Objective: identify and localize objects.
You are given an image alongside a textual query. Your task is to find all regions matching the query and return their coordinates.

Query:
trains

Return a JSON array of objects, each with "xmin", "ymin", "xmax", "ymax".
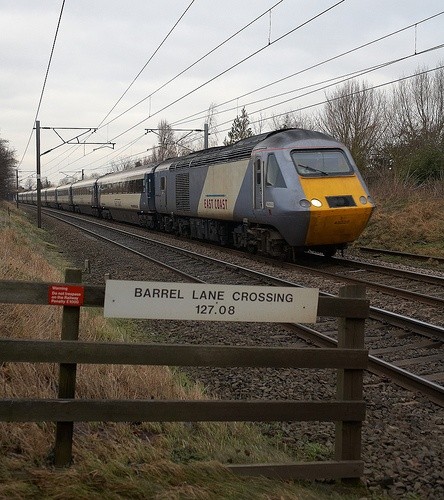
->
[{"xmin": 14, "ymin": 125, "xmax": 374, "ymax": 261}]
